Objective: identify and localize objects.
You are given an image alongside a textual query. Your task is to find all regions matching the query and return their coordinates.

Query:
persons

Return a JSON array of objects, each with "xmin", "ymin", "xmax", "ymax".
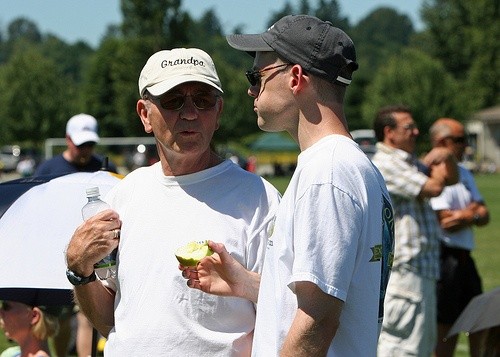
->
[
  {"xmin": 68, "ymin": 288, "xmax": 97, "ymax": 357},
  {"xmin": 66, "ymin": 47, "xmax": 283, "ymax": 357},
  {"xmin": 371, "ymin": 107, "xmax": 459, "ymax": 357},
  {"xmin": 178, "ymin": 14, "xmax": 394, "ymax": 357},
  {"xmin": 34, "ymin": 113, "xmax": 115, "ymax": 175},
  {"xmin": 0, "ymin": 288, "xmax": 62, "ymax": 357},
  {"xmin": 427, "ymin": 117, "xmax": 489, "ymax": 357}
]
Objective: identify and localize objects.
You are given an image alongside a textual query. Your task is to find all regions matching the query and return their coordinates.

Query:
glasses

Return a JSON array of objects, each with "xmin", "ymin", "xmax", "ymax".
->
[
  {"xmin": 244, "ymin": 63, "xmax": 308, "ymax": 86},
  {"xmin": 143, "ymin": 92, "xmax": 220, "ymax": 111}
]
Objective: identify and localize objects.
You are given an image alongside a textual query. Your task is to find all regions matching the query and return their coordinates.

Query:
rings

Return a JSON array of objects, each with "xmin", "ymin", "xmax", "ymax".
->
[{"xmin": 113, "ymin": 229, "xmax": 120, "ymax": 239}]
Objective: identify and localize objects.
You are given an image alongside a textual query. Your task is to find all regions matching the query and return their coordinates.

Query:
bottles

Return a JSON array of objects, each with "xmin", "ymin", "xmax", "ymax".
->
[{"xmin": 81, "ymin": 187, "xmax": 116, "ymax": 280}]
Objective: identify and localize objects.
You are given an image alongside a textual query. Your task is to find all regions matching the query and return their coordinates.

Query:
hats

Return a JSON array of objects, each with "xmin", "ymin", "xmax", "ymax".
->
[
  {"xmin": 226, "ymin": 14, "xmax": 359, "ymax": 86},
  {"xmin": 65, "ymin": 113, "xmax": 100, "ymax": 147},
  {"xmin": 138, "ymin": 47, "xmax": 224, "ymax": 99}
]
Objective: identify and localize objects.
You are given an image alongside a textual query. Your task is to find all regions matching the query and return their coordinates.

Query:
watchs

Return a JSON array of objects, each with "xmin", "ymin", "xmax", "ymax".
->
[
  {"xmin": 473, "ymin": 211, "xmax": 480, "ymax": 225},
  {"xmin": 65, "ymin": 267, "xmax": 96, "ymax": 286}
]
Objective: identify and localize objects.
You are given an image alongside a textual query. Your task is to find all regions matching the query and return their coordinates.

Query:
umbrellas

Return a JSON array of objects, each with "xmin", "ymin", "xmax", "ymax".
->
[
  {"xmin": 0, "ymin": 169, "xmax": 125, "ymax": 288},
  {"xmin": 443, "ymin": 287, "xmax": 500, "ymax": 342}
]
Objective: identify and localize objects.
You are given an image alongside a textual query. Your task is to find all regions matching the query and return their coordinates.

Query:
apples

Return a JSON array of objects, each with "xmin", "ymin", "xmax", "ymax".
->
[{"xmin": 174, "ymin": 242, "xmax": 214, "ymax": 267}]
